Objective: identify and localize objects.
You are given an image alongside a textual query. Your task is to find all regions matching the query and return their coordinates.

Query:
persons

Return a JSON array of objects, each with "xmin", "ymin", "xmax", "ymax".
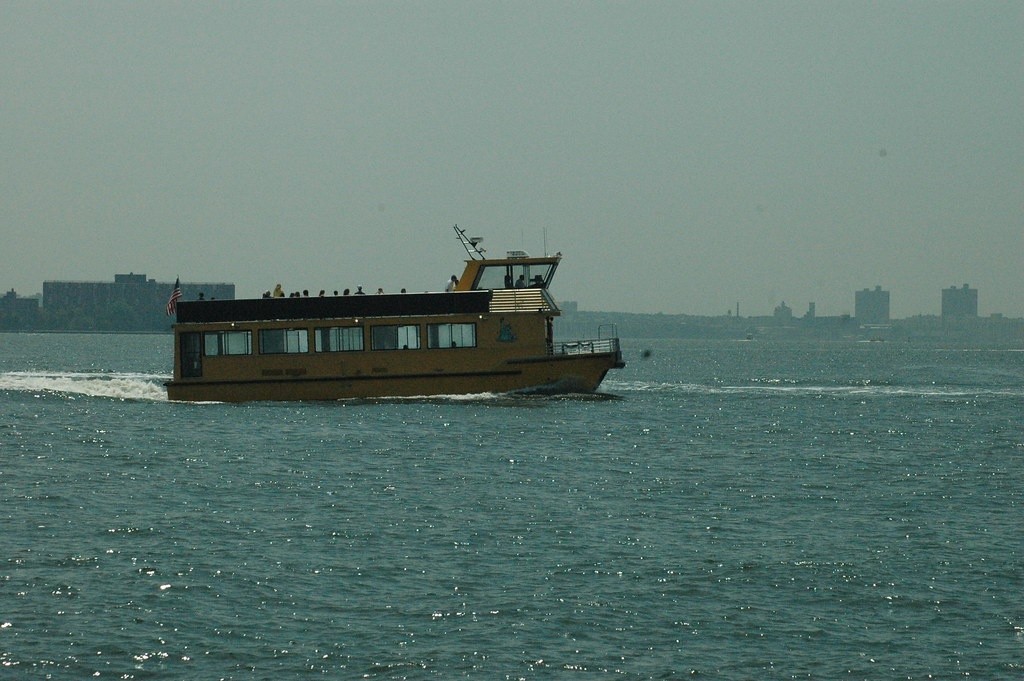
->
[
  {"xmin": 401, "ymin": 288, "xmax": 406, "ymax": 293},
  {"xmin": 515, "ymin": 275, "xmax": 525, "ymax": 288},
  {"xmin": 262, "ymin": 284, "xmax": 285, "ymax": 298},
  {"xmin": 529, "ymin": 275, "xmax": 544, "ymax": 288},
  {"xmin": 303, "ymin": 290, "xmax": 309, "ymax": 297},
  {"xmin": 334, "ymin": 290, "xmax": 338, "ymax": 296},
  {"xmin": 319, "ymin": 290, "xmax": 325, "ymax": 296},
  {"xmin": 289, "ymin": 291, "xmax": 300, "ymax": 298},
  {"xmin": 344, "ymin": 289, "xmax": 350, "ymax": 295},
  {"xmin": 354, "ymin": 286, "xmax": 365, "ymax": 295},
  {"xmin": 375, "ymin": 288, "xmax": 384, "ymax": 294},
  {"xmin": 445, "ymin": 275, "xmax": 459, "ymax": 292},
  {"xmin": 199, "ymin": 292, "xmax": 205, "ymax": 300},
  {"xmin": 505, "ymin": 275, "xmax": 513, "ymax": 288},
  {"xmin": 211, "ymin": 297, "xmax": 215, "ymax": 300},
  {"xmin": 451, "ymin": 341, "xmax": 457, "ymax": 347},
  {"xmin": 403, "ymin": 345, "xmax": 407, "ymax": 349}
]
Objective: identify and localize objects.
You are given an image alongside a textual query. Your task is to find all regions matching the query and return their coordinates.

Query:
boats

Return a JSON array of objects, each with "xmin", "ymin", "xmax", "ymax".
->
[{"xmin": 163, "ymin": 223, "xmax": 625, "ymax": 401}]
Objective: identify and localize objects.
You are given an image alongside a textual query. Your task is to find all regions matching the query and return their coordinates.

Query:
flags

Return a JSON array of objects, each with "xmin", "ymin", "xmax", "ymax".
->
[{"xmin": 166, "ymin": 276, "xmax": 182, "ymax": 316}]
